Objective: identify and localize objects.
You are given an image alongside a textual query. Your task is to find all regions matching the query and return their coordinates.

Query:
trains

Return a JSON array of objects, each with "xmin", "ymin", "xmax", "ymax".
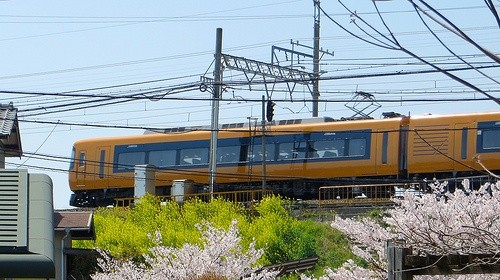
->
[{"xmin": 68, "ymin": 113, "xmax": 500, "ymax": 210}]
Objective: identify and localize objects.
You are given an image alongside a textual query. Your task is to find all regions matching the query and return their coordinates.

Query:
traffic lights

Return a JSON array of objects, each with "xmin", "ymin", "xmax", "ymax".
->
[{"xmin": 267, "ymin": 99, "xmax": 274, "ymax": 122}]
